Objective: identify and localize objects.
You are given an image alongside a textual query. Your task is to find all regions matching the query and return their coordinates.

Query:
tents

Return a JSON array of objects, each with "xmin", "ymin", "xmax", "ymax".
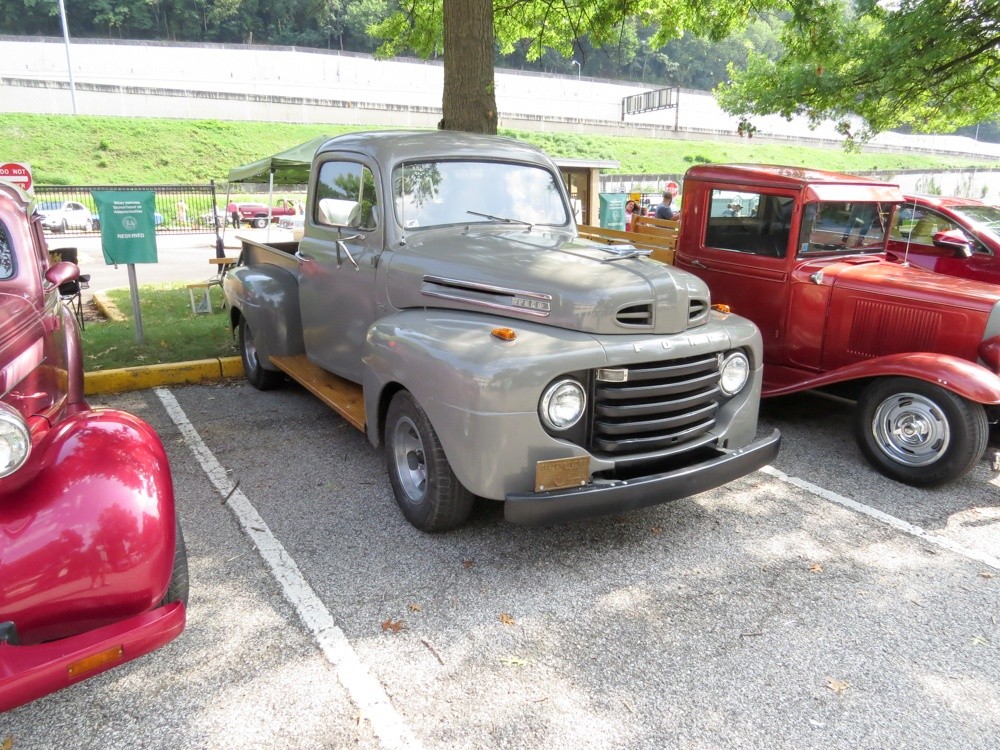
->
[{"xmin": 223, "ymin": 134, "xmax": 332, "ymax": 243}]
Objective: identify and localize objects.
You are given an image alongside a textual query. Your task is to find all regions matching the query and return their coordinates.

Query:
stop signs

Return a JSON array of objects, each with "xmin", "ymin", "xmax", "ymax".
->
[
  {"xmin": 0, "ymin": 161, "xmax": 35, "ymax": 196},
  {"xmin": 665, "ymin": 182, "xmax": 678, "ymax": 197}
]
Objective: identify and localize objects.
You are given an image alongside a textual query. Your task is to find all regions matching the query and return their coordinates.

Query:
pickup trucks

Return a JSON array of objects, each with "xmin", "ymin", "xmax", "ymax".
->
[
  {"xmin": 239, "ymin": 199, "xmax": 305, "ymax": 228},
  {"xmin": 223, "ymin": 129, "xmax": 782, "ymax": 537},
  {"xmin": 575, "ymin": 165, "xmax": 1000, "ymax": 491}
]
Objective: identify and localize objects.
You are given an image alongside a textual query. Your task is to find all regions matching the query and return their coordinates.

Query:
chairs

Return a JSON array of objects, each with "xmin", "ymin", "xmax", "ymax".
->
[
  {"xmin": 186, "ymin": 255, "xmax": 242, "ymax": 315},
  {"xmin": 48, "ymin": 248, "xmax": 90, "ymax": 331}
]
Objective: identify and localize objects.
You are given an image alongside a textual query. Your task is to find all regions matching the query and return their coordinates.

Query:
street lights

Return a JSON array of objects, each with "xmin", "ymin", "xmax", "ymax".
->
[{"xmin": 571, "ymin": 59, "xmax": 581, "ymax": 81}]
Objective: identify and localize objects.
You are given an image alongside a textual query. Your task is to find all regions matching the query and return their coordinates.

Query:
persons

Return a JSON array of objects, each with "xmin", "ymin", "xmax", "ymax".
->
[
  {"xmin": 625, "ymin": 191, "xmax": 681, "ymax": 232},
  {"xmin": 213, "ymin": 199, "xmax": 220, "ymax": 222},
  {"xmin": 228, "ymin": 200, "xmax": 240, "ymax": 229},
  {"xmin": 721, "ymin": 196, "xmax": 758, "ymax": 217},
  {"xmin": 175, "ymin": 198, "xmax": 189, "ymax": 223},
  {"xmin": 831, "ymin": 201, "xmax": 876, "ymax": 248}
]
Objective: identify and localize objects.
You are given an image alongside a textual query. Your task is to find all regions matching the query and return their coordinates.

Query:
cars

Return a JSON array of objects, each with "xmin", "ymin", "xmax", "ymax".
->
[
  {"xmin": 197, "ymin": 202, "xmax": 265, "ymax": 228},
  {"xmin": 886, "ymin": 195, "xmax": 1000, "ymax": 286},
  {"xmin": 0, "ymin": 184, "xmax": 193, "ymax": 717},
  {"xmin": 34, "ymin": 201, "xmax": 93, "ymax": 233}
]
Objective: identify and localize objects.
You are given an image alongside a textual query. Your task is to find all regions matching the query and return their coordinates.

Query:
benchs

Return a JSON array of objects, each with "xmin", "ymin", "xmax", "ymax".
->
[{"xmin": 709, "ymin": 227, "xmax": 801, "ymax": 257}]
